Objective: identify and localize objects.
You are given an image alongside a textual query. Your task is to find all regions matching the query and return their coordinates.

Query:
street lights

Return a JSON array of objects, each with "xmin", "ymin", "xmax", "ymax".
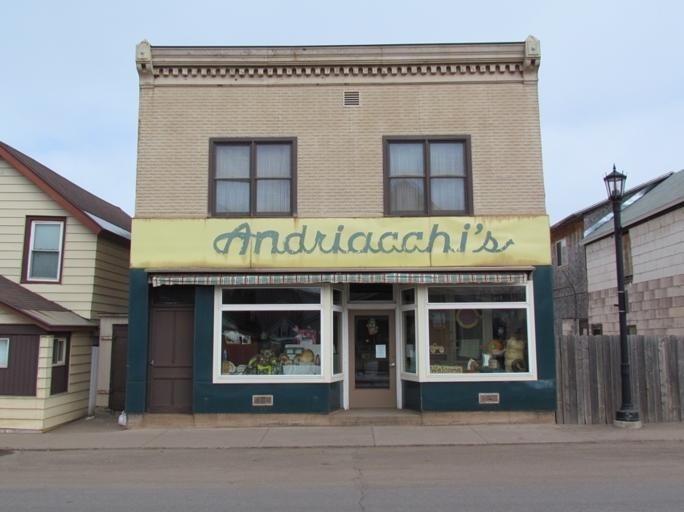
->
[{"xmin": 603, "ymin": 164, "xmax": 641, "ymax": 430}]
